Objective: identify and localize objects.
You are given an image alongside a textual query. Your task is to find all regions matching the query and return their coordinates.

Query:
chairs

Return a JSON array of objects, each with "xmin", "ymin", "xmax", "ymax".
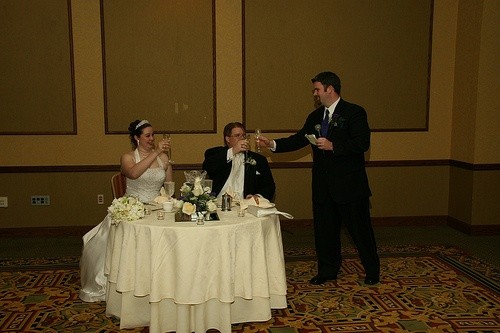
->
[{"xmin": 111, "ymin": 172, "xmax": 125, "ymax": 199}]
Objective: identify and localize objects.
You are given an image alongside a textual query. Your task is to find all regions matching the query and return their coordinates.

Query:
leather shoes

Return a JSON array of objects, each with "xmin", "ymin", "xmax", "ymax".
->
[
  {"xmin": 309, "ymin": 274, "xmax": 338, "ymax": 285},
  {"xmin": 364, "ymin": 272, "xmax": 379, "ymax": 285}
]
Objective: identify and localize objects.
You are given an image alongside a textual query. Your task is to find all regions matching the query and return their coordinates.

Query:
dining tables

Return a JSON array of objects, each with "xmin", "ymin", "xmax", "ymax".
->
[{"xmin": 102, "ymin": 194, "xmax": 288, "ymax": 333}]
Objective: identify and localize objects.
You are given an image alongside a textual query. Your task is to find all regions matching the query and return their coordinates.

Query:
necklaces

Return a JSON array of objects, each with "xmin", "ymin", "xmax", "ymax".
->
[{"xmin": 137, "ymin": 149, "xmax": 148, "ymax": 158}]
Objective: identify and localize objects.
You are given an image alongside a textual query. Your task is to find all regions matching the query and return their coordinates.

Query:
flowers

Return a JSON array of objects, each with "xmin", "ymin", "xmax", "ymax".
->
[
  {"xmin": 106, "ymin": 194, "xmax": 147, "ymax": 226},
  {"xmin": 175, "ymin": 178, "xmax": 217, "ymax": 216}
]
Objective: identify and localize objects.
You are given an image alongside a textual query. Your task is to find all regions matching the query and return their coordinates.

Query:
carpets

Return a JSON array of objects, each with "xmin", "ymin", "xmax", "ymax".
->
[{"xmin": 0, "ymin": 244, "xmax": 500, "ymax": 333}]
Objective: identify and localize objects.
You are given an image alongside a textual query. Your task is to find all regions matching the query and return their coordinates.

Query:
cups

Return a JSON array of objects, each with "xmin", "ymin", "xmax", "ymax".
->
[
  {"xmin": 222, "ymin": 193, "xmax": 232, "ymax": 212},
  {"xmin": 144, "ymin": 206, "xmax": 152, "ymax": 215},
  {"xmin": 238, "ymin": 209, "xmax": 245, "ymax": 217},
  {"xmin": 254, "ymin": 128, "xmax": 263, "ymax": 154},
  {"xmin": 163, "ymin": 202, "xmax": 173, "ymax": 211},
  {"xmin": 156, "ymin": 211, "xmax": 165, "ymax": 220},
  {"xmin": 202, "ymin": 180, "xmax": 213, "ymax": 192},
  {"xmin": 196, "ymin": 218, "xmax": 203, "ymax": 225}
]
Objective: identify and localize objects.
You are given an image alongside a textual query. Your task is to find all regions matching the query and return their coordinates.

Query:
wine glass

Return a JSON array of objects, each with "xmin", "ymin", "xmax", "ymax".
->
[
  {"xmin": 164, "ymin": 182, "xmax": 175, "ymax": 201},
  {"xmin": 239, "ymin": 134, "xmax": 253, "ymax": 164},
  {"xmin": 163, "ymin": 133, "xmax": 175, "ymax": 164}
]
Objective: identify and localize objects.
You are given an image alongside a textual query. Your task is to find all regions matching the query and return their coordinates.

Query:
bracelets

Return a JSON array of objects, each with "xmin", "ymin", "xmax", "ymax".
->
[{"xmin": 148, "ymin": 148, "xmax": 158, "ymax": 161}]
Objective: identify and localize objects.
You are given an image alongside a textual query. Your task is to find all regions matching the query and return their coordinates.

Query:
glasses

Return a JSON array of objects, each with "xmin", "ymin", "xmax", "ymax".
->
[{"xmin": 230, "ymin": 135, "xmax": 247, "ymax": 140}]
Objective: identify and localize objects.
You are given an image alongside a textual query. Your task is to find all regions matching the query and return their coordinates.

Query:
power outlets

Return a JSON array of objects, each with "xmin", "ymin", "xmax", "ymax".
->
[
  {"xmin": 97, "ymin": 194, "xmax": 104, "ymax": 205},
  {"xmin": 30, "ymin": 195, "xmax": 50, "ymax": 205},
  {"xmin": 0, "ymin": 197, "xmax": 9, "ymax": 208}
]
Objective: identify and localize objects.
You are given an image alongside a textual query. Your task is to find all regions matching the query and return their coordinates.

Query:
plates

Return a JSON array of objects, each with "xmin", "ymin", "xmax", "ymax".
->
[{"xmin": 158, "ymin": 210, "xmax": 177, "ymax": 213}]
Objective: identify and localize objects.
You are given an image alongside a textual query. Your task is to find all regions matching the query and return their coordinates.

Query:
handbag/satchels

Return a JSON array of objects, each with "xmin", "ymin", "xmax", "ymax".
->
[{"xmin": 248, "ymin": 204, "xmax": 296, "ymax": 220}]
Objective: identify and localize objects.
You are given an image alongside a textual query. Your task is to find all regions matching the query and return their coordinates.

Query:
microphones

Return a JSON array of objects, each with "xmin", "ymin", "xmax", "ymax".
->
[{"xmin": 314, "ymin": 124, "xmax": 326, "ymax": 154}]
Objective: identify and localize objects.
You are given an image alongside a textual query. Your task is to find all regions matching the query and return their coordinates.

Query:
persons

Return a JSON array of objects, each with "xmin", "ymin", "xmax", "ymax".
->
[
  {"xmin": 120, "ymin": 119, "xmax": 172, "ymax": 202},
  {"xmin": 202, "ymin": 122, "xmax": 276, "ymax": 202},
  {"xmin": 256, "ymin": 72, "xmax": 378, "ymax": 285}
]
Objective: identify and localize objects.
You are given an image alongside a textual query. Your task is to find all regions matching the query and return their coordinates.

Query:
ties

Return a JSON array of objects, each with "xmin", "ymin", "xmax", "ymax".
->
[{"xmin": 322, "ymin": 109, "xmax": 329, "ymax": 136}]
